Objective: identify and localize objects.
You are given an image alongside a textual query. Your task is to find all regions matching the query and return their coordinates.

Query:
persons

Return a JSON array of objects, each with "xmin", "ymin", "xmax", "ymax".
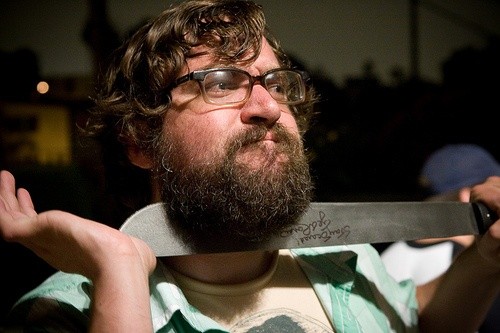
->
[
  {"xmin": 380, "ymin": 144, "xmax": 500, "ymax": 333},
  {"xmin": 0, "ymin": 0, "xmax": 500, "ymax": 333}
]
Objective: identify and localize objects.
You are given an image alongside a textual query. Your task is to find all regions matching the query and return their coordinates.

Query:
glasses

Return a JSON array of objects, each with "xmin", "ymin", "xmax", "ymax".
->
[{"xmin": 158, "ymin": 67, "xmax": 307, "ymax": 104}]
